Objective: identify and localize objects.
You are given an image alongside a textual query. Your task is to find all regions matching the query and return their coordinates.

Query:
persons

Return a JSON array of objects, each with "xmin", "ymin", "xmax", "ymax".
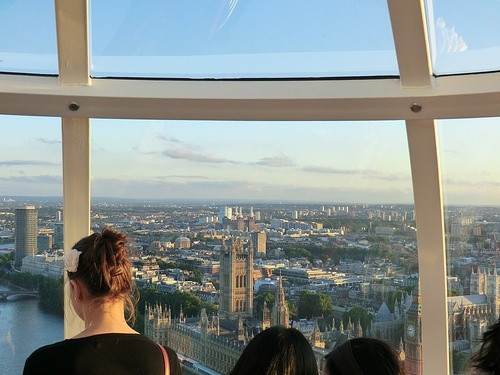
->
[
  {"xmin": 323, "ymin": 336, "xmax": 405, "ymax": 374},
  {"xmin": 21, "ymin": 227, "xmax": 183, "ymax": 375},
  {"xmin": 229, "ymin": 325, "xmax": 320, "ymax": 374}
]
